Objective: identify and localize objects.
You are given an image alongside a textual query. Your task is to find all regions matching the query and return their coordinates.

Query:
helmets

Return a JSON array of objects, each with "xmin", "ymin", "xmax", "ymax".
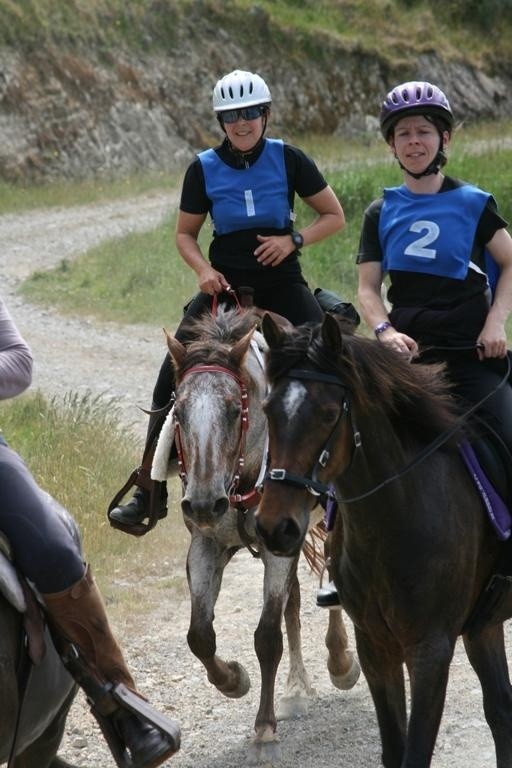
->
[
  {"xmin": 380, "ymin": 80, "xmax": 454, "ymax": 144},
  {"xmin": 213, "ymin": 70, "xmax": 272, "ymax": 112}
]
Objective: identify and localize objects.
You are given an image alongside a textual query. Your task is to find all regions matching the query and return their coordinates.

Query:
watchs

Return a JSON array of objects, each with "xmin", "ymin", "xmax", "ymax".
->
[
  {"xmin": 374, "ymin": 322, "xmax": 393, "ymax": 336},
  {"xmin": 289, "ymin": 231, "xmax": 304, "ymax": 251}
]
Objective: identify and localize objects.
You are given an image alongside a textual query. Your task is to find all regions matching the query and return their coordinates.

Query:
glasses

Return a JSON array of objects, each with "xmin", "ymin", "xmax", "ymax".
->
[{"xmin": 222, "ymin": 108, "xmax": 266, "ymax": 123}]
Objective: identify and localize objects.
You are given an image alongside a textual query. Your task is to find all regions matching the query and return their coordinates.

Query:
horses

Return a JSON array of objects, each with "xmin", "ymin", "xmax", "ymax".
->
[
  {"xmin": 253, "ymin": 310, "xmax": 511, "ymax": 768},
  {"xmin": 0, "ymin": 515, "xmax": 83, "ymax": 767},
  {"xmin": 153, "ymin": 301, "xmax": 366, "ymax": 768}
]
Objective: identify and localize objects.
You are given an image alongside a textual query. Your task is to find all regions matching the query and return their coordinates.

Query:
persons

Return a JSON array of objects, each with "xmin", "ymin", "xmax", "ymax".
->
[
  {"xmin": 0, "ymin": 296, "xmax": 182, "ymax": 768},
  {"xmin": 315, "ymin": 82, "xmax": 512, "ymax": 612},
  {"xmin": 107, "ymin": 70, "xmax": 344, "ymax": 536}
]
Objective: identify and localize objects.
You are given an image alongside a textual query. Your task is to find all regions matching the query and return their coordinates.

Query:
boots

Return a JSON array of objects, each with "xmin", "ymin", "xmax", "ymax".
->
[
  {"xmin": 43, "ymin": 560, "xmax": 173, "ymax": 767},
  {"xmin": 315, "ymin": 580, "xmax": 341, "ymax": 607},
  {"xmin": 109, "ymin": 401, "xmax": 175, "ymax": 525}
]
